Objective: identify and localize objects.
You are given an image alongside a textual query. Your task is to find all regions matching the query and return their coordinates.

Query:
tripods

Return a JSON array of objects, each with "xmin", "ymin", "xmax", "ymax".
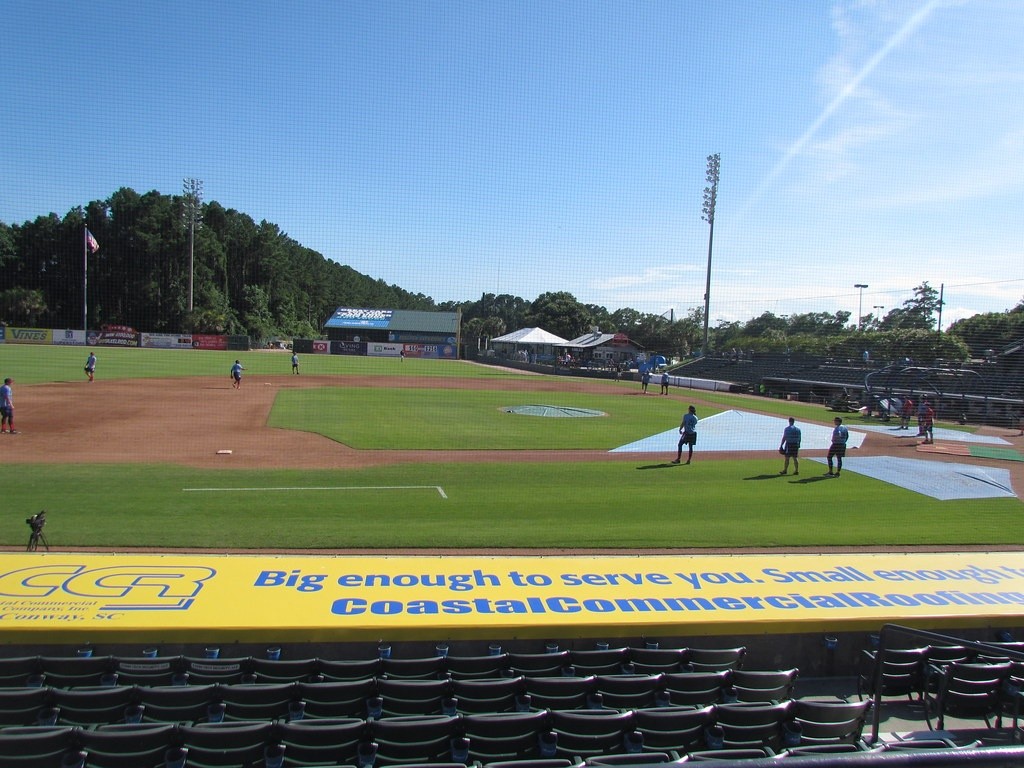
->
[{"xmin": 26, "ymin": 532, "xmax": 49, "ymax": 552}]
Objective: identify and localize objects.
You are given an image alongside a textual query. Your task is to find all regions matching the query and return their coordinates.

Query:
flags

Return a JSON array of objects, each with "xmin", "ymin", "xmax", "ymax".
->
[{"xmin": 87, "ymin": 230, "xmax": 99, "ymax": 253}]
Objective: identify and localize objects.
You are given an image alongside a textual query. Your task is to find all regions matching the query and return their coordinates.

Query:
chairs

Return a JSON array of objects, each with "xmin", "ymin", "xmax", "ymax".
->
[{"xmin": 0, "ymin": 642, "xmax": 1024, "ymax": 768}]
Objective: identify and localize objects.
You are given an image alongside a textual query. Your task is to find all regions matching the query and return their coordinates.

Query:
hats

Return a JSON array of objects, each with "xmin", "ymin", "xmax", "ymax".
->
[{"xmin": 832, "ymin": 416, "xmax": 843, "ymax": 423}]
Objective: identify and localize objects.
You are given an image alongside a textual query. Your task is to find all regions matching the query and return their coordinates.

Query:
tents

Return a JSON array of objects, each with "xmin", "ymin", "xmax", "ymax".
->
[{"xmin": 490, "ymin": 327, "xmax": 570, "ymax": 359}]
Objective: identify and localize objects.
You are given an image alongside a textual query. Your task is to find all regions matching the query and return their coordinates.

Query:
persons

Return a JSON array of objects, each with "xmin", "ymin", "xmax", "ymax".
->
[
  {"xmin": 615, "ymin": 365, "xmax": 623, "ymax": 382},
  {"xmin": 918, "ymin": 393, "xmax": 934, "ymax": 444},
  {"xmin": 898, "ymin": 395, "xmax": 913, "ymax": 430},
  {"xmin": 497, "ymin": 350, "xmax": 629, "ymax": 371},
  {"xmin": 725, "ymin": 347, "xmax": 744, "ymax": 364},
  {"xmin": 84, "ymin": 352, "xmax": 96, "ymax": 383},
  {"xmin": 863, "ymin": 347, "xmax": 869, "ymax": 369},
  {"xmin": 231, "ymin": 360, "xmax": 248, "ymax": 389},
  {"xmin": 642, "ymin": 370, "xmax": 652, "ymax": 394},
  {"xmin": 754, "ymin": 384, "xmax": 759, "ymax": 395},
  {"xmin": 292, "ymin": 351, "xmax": 299, "ymax": 374},
  {"xmin": 824, "ymin": 417, "xmax": 848, "ymax": 477},
  {"xmin": 779, "ymin": 417, "xmax": 801, "ymax": 475},
  {"xmin": 660, "ymin": 370, "xmax": 669, "ymax": 395},
  {"xmin": 671, "ymin": 406, "xmax": 698, "ymax": 464},
  {"xmin": 400, "ymin": 349, "xmax": 405, "ymax": 361},
  {"xmin": 759, "ymin": 383, "xmax": 765, "ymax": 397},
  {"xmin": 0, "ymin": 377, "xmax": 21, "ymax": 434}
]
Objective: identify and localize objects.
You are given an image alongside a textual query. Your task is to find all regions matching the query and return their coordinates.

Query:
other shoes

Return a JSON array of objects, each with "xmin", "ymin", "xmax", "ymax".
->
[
  {"xmin": 685, "ymin": 460, "xmax": 690, "ymax": 464},
  {"xmin": 236, "ymin": 386, "xmax": 241, "ymax": 390},
  {"xmin": 671, "ymin": 459, "xmax": 681, "ymax": 464},
  {"xmin": 780, "ymin": 471, "xmax": 787, "ymax": 474},
  {"xmin": 10, "ymin": 430, "xmax": 21, "ymax": 434},
  {"xmin": 905, "ymin": 427, "xmax": 908, "ymax": 430},
  {"xmin": 644, "ymin": 391, "xmax": 648, "ymax": 394},
  {"xmin": 922, "ymin": 439, "xmax": 928, "ymax": 443},
  {"xmin": 927, "ymin": 441, "xmax": 933, "ymax": 445},
  {"xmin": 823, "ymin": 472, "xmax": 832, "ymax": 476},
  {"xmin": 232, "ymin": 383, "xmax": 236, "ymax": 387},
  {"xmin": 88, "ymin": 379, "xmax": 94, "ymax": 381},
  {"xmin": 2, "ymin": 429, "xmax": 9, "ymax": 432},
  {"xmin": 898, "ymin": 427, "xmax": 903, "ymax": 429},
  {"xmin": 660, "ymin": 393, "xmax": 663, "ymax": 394},
  {"xmin": 792, "ymin": 472, "xmax": 799, "ymax": 475},
  {"xmin": 665, "ymin": 393, "xmax": 668, "ymax": 396},
  {"xmin": 834, "ymin": 472, "xmax": 840, "ymax": 477},
  {"xmin": 297, "ymin": 372, "xmax": 299, "ymax": 375},
  {"xmin": 918, "ymin": 433, "xmax": 925, "ymax": 436}
]
